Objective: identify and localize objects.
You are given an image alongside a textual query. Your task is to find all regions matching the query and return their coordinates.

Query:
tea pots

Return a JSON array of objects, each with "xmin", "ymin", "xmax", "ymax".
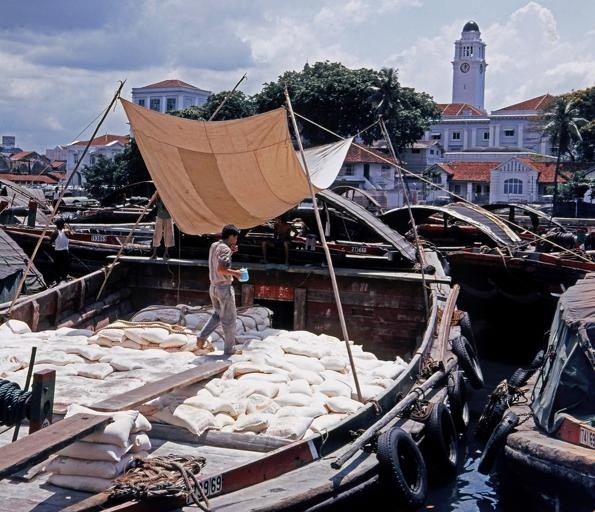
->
[{"xmin": 237, "ymin": 268, "xmax": 251, "ymax": 283}]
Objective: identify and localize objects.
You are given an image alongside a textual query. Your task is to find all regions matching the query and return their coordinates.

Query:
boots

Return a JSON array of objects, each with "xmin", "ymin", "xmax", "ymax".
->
[
  {"xmin": 163, "ymin": 247, "xmax": 169, "ymax": 260},
  {"xmin": 150, "ymin": 247, "xmax": 158, "ymax": 260}
]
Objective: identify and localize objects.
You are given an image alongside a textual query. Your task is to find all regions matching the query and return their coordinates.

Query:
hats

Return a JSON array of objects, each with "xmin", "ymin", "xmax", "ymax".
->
[{"xmin": 54, "ymin": 218, "xmax": 65, "ymax": 224}]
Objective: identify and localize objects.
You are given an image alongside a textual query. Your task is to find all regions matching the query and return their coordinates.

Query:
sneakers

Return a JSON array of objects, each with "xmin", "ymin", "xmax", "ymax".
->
[{"xmin": 224, "ymin": 351, "xmax": 242, "ymax": 354}]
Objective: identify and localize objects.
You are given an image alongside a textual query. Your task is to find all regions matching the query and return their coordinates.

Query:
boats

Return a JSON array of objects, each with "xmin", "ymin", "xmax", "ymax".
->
[
  {"xmin": 0, "ymin": 184, "xmax": 461, "ymax": 512},
  {"xmin": 501, "ymin": 277, "xmax": 595, "ymax": 489}
]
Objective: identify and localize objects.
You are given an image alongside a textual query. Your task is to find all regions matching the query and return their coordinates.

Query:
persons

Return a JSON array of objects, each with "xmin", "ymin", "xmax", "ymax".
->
[
  {"xmin": 148, "ymin": 191, "xmax": 176, "ymax": 262},
  {"xmin": 49, "ymin": 217, "xmax": 72, "ymax": 284},
  {"xmin": 259, "ymin": 214, "xmax": 299, "ymax": 267},
  {"xmin": 26, "ymin": 196, "xmax": 38, "ymax": 228},
  {"xmin": 196, "ymin": 224, "xmax": 244, "ymax": 356}
]
